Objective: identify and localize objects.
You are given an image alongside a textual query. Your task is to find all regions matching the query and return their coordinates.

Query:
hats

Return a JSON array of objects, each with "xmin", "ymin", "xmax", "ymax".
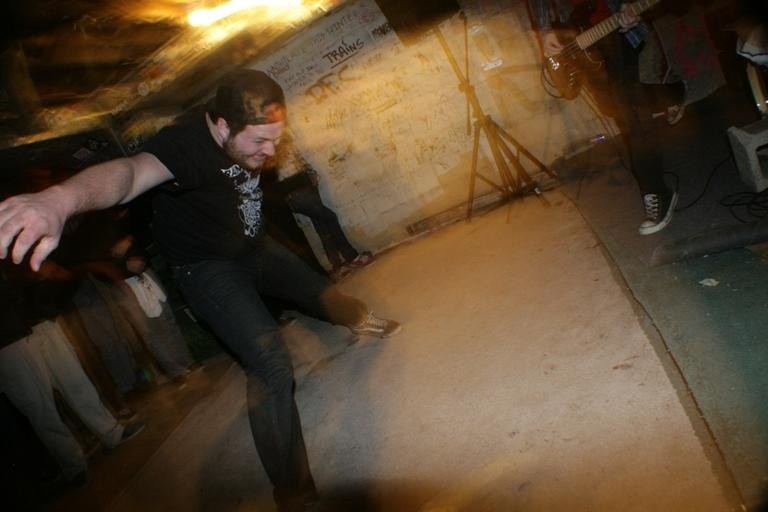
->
[{"xmin": 208, "ymin": 70, "xmax": 286, "ymax": 124}]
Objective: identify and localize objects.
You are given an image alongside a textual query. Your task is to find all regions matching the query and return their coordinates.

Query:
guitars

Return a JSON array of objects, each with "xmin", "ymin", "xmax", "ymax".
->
[{"xmin": 544, "ymin": 0, "xmax": 657, "ymax": 99}]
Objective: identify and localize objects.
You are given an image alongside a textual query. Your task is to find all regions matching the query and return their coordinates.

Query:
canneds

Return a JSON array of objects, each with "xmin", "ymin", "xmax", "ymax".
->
[{"xmin": 590, "ymin": 135, "xmax": 606, "ymax": 142}]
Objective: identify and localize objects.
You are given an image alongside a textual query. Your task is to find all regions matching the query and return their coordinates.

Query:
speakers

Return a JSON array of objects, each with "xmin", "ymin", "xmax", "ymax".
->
[{"xmin": 375, "ymin": 0, "xmax": 461, "ymax": 48}]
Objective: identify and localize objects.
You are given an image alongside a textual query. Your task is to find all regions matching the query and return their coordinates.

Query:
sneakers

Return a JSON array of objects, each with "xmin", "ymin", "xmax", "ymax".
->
[
  {"xmin": 120, "ymin": 425, "xmax": 145, "ymax": 444},
  {"xmin": 118, "ymin": 411, "xmax": 138, "ymax": 423},
  {"xmin": 277, "ymin": 492, "xmax": 328, "ymax": 511},
  {"xmin": 275, "ymin": 311, "xmax": 297, "ymax": 326},
  {"xmin": 335, "ymin": 266, "xmax": 353, "ymax": 280},
  {"xmin": 638, "ymin": 192, "xmax": 679, "ymax": 235},
  {"xmin": 349, "ymin": 254, "xmax": 376, "ymax": 268},
  {"xmin": 351, "ymin": 311, "xmax": 402, "ymax": 338},
  {"xmin": 666, "ymin": 80, "xmax": 689, "ymax": 124}
]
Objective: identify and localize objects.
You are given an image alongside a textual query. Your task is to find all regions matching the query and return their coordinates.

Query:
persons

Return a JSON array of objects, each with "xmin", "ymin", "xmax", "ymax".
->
[
  {"xmin": 526, "ymin": 1, "xmax": 687, "ymax": 237},
  {"xmin": 1, "ymin": 69, "xmax": 404, "ymax": 512}
]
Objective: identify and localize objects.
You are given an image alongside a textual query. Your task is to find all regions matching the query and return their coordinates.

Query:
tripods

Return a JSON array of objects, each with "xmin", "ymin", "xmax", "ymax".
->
[{"xmin": 458, "ymin": 71, "xmax": 564, "ymax": 224}]
